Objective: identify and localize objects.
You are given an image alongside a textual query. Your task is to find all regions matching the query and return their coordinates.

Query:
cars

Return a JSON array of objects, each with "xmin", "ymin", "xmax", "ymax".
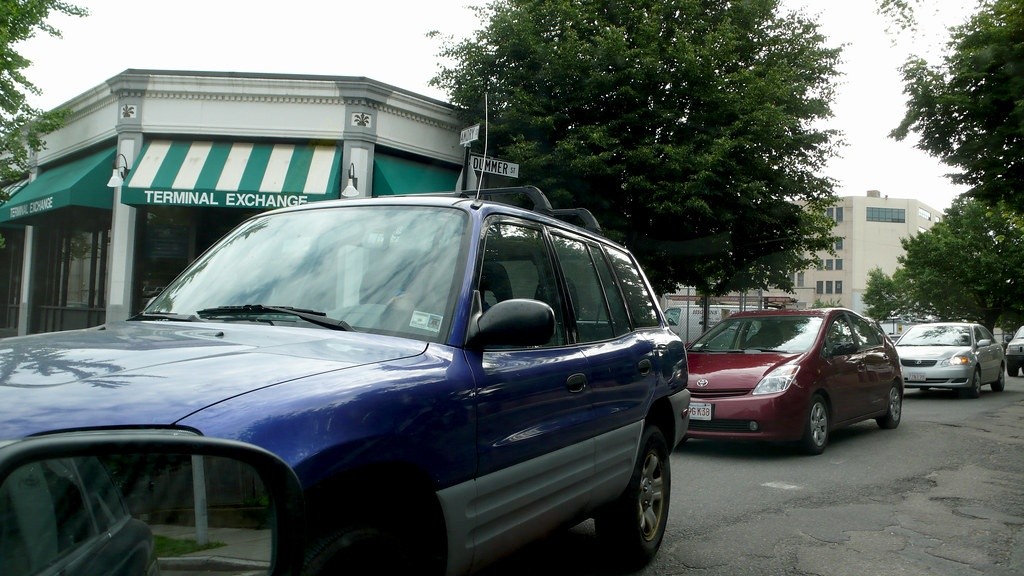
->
[
  {"xmin": 1007, "ymin": 326, "xmax": 1024, "ymax": 377},
  {"xmin": 893, "ymin": 321, "xmax": 1007, "ymax": 398},
  {"xmin": 678, "ymin": 307, "xmax": 905, "ymax": 456}
]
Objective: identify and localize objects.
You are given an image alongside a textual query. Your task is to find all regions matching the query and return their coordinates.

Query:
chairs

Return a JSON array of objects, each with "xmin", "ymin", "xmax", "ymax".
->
[
  {"xmin": 476, "ymin": 261, "xmax": 513, "ymax": 315},
  {"xmin": 534, "ymin": 278, "xmax": 580, "ymax": 350},
  {"xmin": 743, "ymin": 324, "xmax": 783, "ymax": 350}
]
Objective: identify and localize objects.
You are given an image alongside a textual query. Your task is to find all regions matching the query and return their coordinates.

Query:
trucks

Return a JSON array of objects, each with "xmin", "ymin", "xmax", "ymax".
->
[{"xmin": 658, "ymin": 289, "xmax": 800, "ymax": 352}]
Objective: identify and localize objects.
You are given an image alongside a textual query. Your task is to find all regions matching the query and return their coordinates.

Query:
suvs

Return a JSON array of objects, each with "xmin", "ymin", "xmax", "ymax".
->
[{"xmin": 0, "ymin": 183, "xmax": 692, "ymax": 576}]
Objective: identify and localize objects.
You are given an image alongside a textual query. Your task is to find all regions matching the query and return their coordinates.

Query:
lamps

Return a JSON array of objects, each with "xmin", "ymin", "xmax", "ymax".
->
[
  {"xmin": 342, "ymin": 162, "xmax": 359, "ymax": 197},
  {"xmin": 106, "ymin": 153, "xmax": 131, "ymax": 188}
]
